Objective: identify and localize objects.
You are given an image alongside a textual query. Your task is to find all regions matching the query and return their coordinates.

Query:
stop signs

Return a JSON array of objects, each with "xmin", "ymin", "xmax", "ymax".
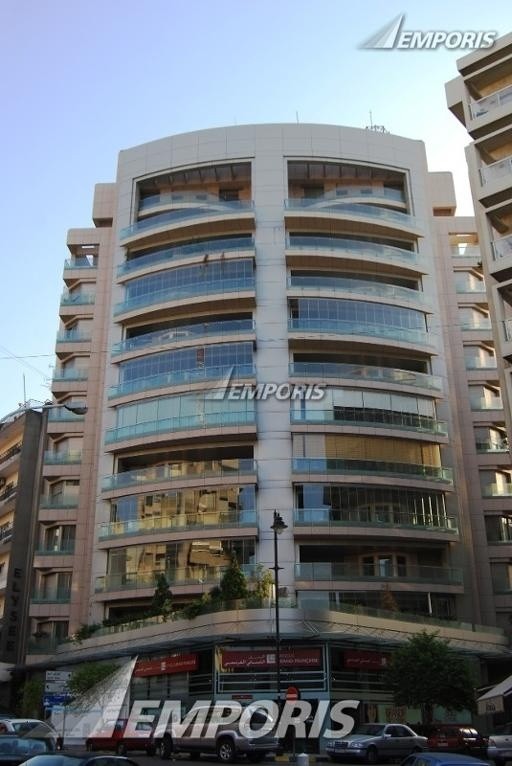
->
[{"xmin": 285, "ymin": 686, "xmax": 299, "ymax": 700}]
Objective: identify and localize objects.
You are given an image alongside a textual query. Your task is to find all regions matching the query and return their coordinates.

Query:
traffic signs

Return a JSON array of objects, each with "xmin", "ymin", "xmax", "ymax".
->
[
  {"xmin": 44, "ymin": 683, "xmax": 71, "ymax": 692},
  {"xmin": 46, "ymin": 671, "xmax": 73, "ymax": 681},
  {"xmin": 43, "ymin": 696, "xmax": 74, "ymax": 705}
]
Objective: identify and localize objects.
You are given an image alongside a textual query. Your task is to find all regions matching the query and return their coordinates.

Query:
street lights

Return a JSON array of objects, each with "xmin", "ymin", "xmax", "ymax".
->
[{"xmin": 269, "ymin": 508, "xmax": 288, "ymax": 699}]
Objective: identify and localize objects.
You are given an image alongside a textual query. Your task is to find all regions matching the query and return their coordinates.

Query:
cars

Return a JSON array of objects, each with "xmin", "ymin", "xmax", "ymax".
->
[{"xmin": 0, "ymin": 717, "xmax": 512, "ymax": 766}]
